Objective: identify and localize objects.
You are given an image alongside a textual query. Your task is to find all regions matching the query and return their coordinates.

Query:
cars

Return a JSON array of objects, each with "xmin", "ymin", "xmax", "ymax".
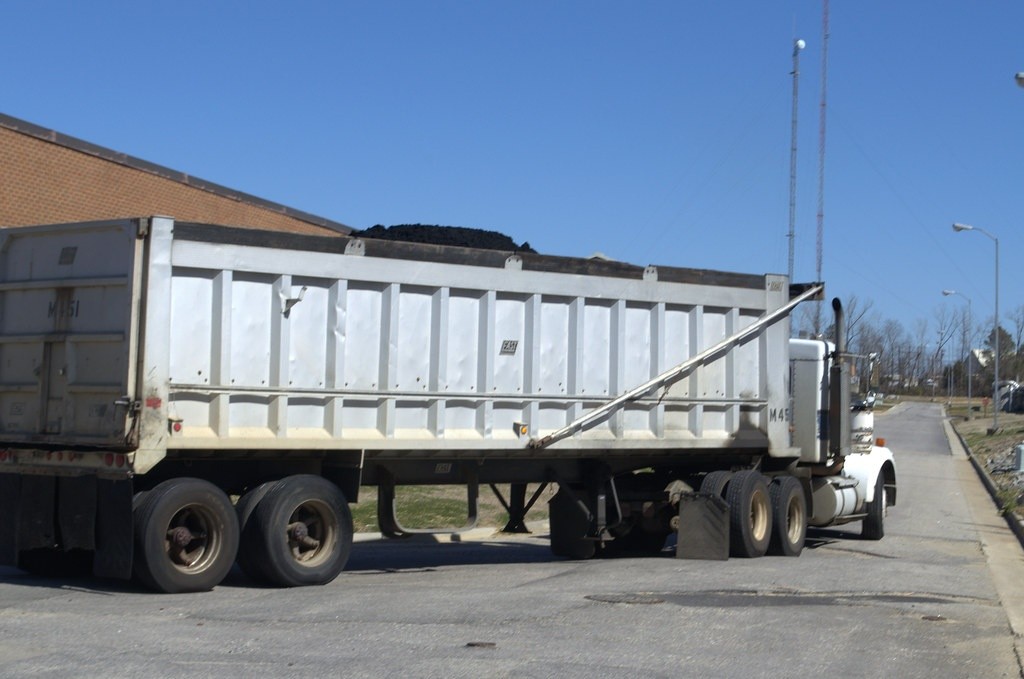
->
[{"xmin": 850, "ymin": 391, "xmax": 877, "ymax": 410}]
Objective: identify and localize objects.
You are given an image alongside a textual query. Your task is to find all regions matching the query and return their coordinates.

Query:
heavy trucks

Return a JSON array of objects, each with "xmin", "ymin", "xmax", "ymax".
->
[{"xmin": 0, "ymin": 212, "xmax": 901, "ymax": 595}]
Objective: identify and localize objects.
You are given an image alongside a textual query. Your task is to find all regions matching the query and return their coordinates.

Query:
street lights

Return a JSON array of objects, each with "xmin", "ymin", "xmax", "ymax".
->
[
  {"xmin": 943, "ymin": 290, "xmax": 973, "ymax": 421},
  {"xmin": 953, "ymin": 222, "xmax": 999, "ymax": 425},
  {"xmin": 787, "ymin": 38, "xmax": 806, "ymax": 284}
]
